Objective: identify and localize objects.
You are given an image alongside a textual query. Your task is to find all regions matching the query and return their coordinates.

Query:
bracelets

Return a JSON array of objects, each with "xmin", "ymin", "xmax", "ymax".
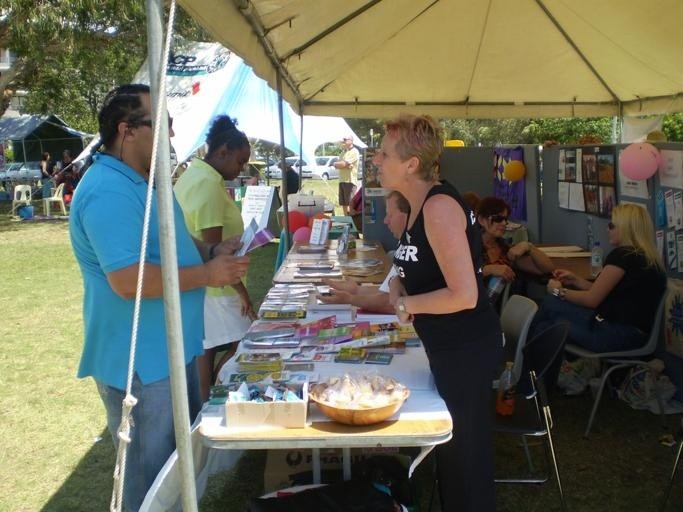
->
[
  {"xmin": 528, "ymin": 241, "xmax": 533, "ymax": 252},
  {"xmin": 560, "ymin": 288, "xmax": 570, "ymax": 300}
]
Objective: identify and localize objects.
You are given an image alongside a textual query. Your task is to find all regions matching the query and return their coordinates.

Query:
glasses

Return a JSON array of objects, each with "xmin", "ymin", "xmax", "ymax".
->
[
  {"xmin": 607, "ymin": 221, "xmax": 616, "ymax": 231},
  {"xmin": 490, "ymin": 215, "xmax": 508, "ymax": 223},
  {"xmin": 343, "ymin": 137, "xmax": 352, "ymax": 142},
  {"xmin": 128, "ymin": 114, "xmax": 173, "ymax": 130}
]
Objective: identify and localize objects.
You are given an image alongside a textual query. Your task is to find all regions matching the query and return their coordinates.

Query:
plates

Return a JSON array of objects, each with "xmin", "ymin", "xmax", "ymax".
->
[{"xmin": 308, "ymin": 376, "xmax": 412, "ymax": 428}]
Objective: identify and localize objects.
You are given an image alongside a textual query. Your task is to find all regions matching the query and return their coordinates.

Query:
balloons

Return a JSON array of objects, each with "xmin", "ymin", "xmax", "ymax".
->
[
  {"xmin": 503, "ymin": 159, "xmax": 525, "ymax": 183},
  {"xmin": 619, "ymin": 143, "xmax": 661, "ymax": 181}
]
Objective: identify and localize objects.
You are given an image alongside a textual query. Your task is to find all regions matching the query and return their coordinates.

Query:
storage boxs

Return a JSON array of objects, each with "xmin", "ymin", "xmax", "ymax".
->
[
  {"xmin": 287, "ymin": 191, "xmax": 326, "ymax": 217},
  {"xmin": 538, "ymin": 244, "xmax": 592, "ymax": 280}
]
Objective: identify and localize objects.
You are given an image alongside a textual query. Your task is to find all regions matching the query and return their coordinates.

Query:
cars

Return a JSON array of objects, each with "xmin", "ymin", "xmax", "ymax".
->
[
  {"xmin": 0, "ymin": 162, "xmax": 42, "ymax": 187},
  {"xmin": 297, "ymin": 156, "xmax": 339, "ymax": 182},
  {"xmin": 264, "ymin": 157, "xmax": 309, "ymax": 180}
]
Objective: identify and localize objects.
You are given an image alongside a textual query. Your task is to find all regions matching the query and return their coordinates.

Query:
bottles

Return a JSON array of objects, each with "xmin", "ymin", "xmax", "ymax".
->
[
  {"xmin": 592, "ymin": 242, "xmax": 602, "ymax": 277},
  {"xmin": 486, "ymin": 261, "xmax": 515, "ymax": 306}
]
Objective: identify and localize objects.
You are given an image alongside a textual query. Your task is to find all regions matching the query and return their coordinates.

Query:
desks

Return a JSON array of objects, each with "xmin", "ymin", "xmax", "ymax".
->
[
  {"xmin": 198, "ymin": 282, "xmax": 454, "ymax": 488},
  {"xmin": 272, "ymin": 240, "xmax": 392, "ymax": 283}
]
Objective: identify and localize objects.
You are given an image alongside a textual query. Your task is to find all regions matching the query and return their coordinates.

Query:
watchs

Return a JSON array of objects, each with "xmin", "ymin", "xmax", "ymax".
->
[
  {"xmin": 209, "ymin": 243, "xmax": 219, "ymax": 259},
  {"xmin": 397, "ymin": 297, "xmax": 407, "ymax": 314},
  {"xmin": 552, "ymin": 288, "xmax": 560, "ymax": 297},
  {"xmin": 477, "ymin": 263, "xmax": 486, "ymax": 275}
]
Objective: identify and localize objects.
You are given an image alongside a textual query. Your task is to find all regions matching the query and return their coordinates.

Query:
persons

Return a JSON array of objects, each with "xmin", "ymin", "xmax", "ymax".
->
[
  {"xmin": 171, "ymin": 113, "xmax": 255, "ymax": 403},
  {"xmin": 278, "ymin": 162, "xmax": 300, "ymax": 194},
  {"xmin": 315, "ymin": 113, "xmax": 506, "ymax": 512},
  {"xmin": 472, "ymin": 195, "xmax": 554, "ymax": 311},
  {"xmin": 314, "ymin": 190, "xmax": 412, "ymax": 314},
  {"xmin": 61, "ymin": 149, "xmax": 74, "ymax": 181},
  {"xmin": 40, "ymin": 152, "xmax": 54, "ymax": 200},
  {"xmin": 70, "ymin": 85, "xmax": 250, "ymax": 512},
  {"xmin": 521, "ymin": 202, "xmax": 667, "ymax": 395},
  {"xmin": 334, "ymin": 135, "xmax": 359, "ymax": 216}
]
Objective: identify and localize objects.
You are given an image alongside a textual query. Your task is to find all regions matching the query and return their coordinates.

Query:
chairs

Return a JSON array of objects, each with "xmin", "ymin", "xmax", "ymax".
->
[
  {"xmin": 537, "ymin": 286, "xmax": 672, "ymax": 441},
  {"xmin": 12, "ymin": 185, "xmax": 32, "ymax": 217},
  {"xmin": 487, "ymin": 293, "xmax": 568, "ymax": 512},
  {"xmin": 42, "ymin": 182, "xmax": 67, "ymax": 217}
]
